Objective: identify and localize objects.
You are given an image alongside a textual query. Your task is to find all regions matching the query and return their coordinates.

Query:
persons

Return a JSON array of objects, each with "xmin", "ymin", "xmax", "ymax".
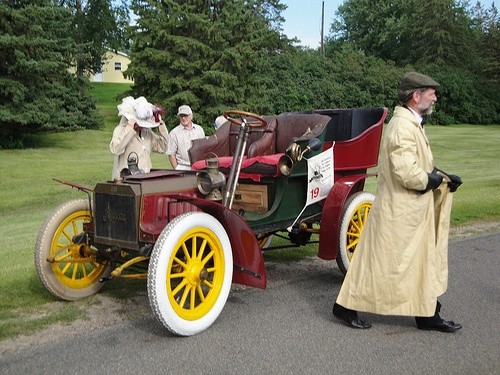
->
[
  {"xmin": 165, "ymin": 105, "xmax": 206, "ymax": 172},
  {"xmin": 332, "ymin": 71, "xmax": 463, "ymax": 333},
  {"xmin": 110, "ymin": 96, "xmax": 170, "ymax": 181}
]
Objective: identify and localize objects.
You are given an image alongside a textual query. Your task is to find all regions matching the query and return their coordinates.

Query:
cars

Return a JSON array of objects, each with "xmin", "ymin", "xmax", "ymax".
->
[{"xmin": 33, "ymin": 106, "xmax": 389, "ymax": 337}]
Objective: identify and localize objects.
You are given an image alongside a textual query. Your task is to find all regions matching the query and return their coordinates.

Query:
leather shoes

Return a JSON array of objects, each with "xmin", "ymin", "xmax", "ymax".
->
[
  {"xmin": 416, "ymin": 315, "xmax": 463, "ymax": 332},
  {"xmin": 333, "ymin": 306, "xmax": 372, "ymax": 329}
]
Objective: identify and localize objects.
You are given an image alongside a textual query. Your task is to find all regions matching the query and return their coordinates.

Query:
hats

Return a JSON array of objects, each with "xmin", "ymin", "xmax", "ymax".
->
[
  {"xmin": 116, "ymin": 96, "xmax": 165, "ymax": 128},
  {"xmin": 399, "ymin": 72, "xmax": 441, "ymax": 88},
  {"xmin": 176, "ymin": 105, "xmax": 193, "ymax": 115}
]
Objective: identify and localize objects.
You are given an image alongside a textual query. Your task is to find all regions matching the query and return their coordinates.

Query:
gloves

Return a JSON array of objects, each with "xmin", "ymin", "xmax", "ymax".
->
[
  {"xmin": 418, "ymin": 173, "xmax": 444, "ymax": 194},
  {"xmin": 432, "ymin": 168, "xmax": 463, "ymax": 192}
]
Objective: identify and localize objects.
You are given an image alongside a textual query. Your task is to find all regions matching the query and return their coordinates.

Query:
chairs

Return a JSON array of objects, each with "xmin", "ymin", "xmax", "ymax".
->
[{"xmin": 187, "ymin": 107, "xmax": 389, "ymax": 176}]
